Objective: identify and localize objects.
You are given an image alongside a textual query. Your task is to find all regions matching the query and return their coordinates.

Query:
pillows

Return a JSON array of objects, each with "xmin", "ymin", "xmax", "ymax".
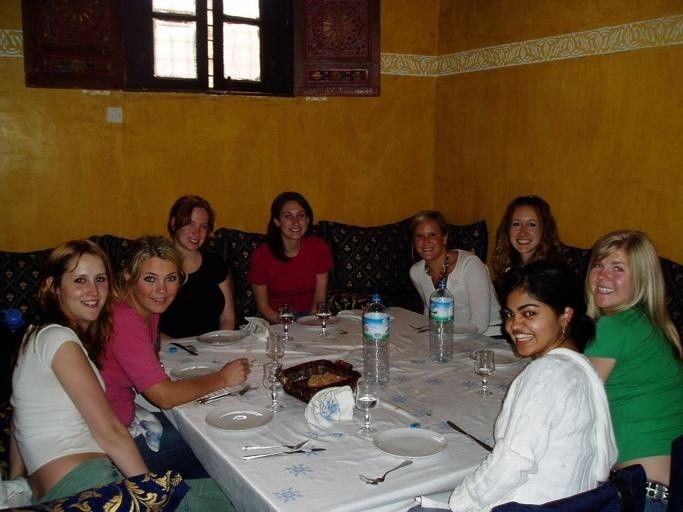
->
[
  {"xmin": 0, "ymin": 467, "xmax": 190, "ymax": 512},
  {"xmin": 227, "ymin": 223, "xmax": 322, "ymax": 317},
  {"xmin": 322, "ymin": 216, "xmax": 415, "ymax": 290},
  {"xmin": 1, "ymin": 235, "xmax": 101, "ymax": 321},
  {"xmin": 103, "ymin": 234, "xmax": 136, "ymax": 276},
  {"xmin": 413, "ymin": 218, "xmax": 488, "ymax": 264}
]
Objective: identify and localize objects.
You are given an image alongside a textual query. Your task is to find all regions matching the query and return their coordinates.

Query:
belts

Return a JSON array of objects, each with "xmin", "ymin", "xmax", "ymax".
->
[{"xmin": 609, "ymin": 468, "xmax": 670, "ymax": 504}]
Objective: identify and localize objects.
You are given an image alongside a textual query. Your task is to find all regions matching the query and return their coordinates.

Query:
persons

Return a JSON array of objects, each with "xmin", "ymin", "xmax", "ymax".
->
[
  {"xmin": 245, "ymin": 190, "xmax": 334, "ymax": 324},
  {"xmin": 157, "ymin": 192, "xmax": 236, "ymax": 341},
  {"xmin": 8, "ymin": 238, "xmax": 237, "ymax": 511},
  {"xmin": 488, "ymin": 193, "xmax": 595, "ymax": 354},
  {"xmin": 449, "ymin": 257, "xmax": 619, "ymax": 511},
  {"xmin": 407, "ymin": 209, "xmax": 504, "ymax": 339},
  {"xmin": 581, "ymin": 228, "xmax": 683, "ymax": 511},
  {"xmin": 97, "ymin": 233, "xmax": 251, "ymax": 481}
]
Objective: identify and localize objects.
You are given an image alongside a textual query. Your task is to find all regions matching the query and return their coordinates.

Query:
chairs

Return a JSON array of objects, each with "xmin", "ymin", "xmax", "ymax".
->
[
  {"xmin": 667, "ymin": 436, "xmax": 682, "ymax": 512},
  {"xmin": 491, "ymin": 464, "xmax": 648, "ymax": 512}
]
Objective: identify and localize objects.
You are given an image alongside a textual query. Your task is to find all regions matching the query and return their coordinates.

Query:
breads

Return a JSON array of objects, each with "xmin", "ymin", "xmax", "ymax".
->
[{"xmin": 307, "ymin": 372, "xmax": 347, "ymax": 387}]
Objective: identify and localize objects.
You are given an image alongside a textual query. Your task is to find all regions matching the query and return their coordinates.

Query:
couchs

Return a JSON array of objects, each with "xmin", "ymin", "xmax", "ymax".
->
[
  {"xmin": 558, "ymin": 241, "xmax": 683, "ymax": 345},
  {"xmin": 0, "ymin": 218, "xmax": 486, "ymax": 512}
]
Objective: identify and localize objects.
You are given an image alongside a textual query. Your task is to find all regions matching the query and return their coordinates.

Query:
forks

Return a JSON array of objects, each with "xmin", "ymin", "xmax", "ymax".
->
[
  {"xmin": 408, "ymin": 324, "xmax": 430, "ymax": 330},
  {"xmin": 358, "ymin": 459, "xmax": 414, "ymax": 486},
  {"xmin": 241, "ymin": 438, "xmax": 314, "ymax": 450},
  {"xmin": 200, "ymin": 382, "xmax": 253, "ymax": 404}
]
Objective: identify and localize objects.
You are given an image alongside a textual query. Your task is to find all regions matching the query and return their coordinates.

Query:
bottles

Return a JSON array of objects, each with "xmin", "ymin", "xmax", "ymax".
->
[
  {"xmin": 362, "ymin": 294, "xmax": 390, "ymax": 386},
  {"xmin": 428, "ymin": 280, "xmax": 454, "ymax": 364}
]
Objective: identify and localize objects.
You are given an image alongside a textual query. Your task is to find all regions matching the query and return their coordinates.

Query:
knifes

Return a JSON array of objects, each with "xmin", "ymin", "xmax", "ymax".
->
[
  {"xmin": 197, "ymin": 386, "xmax": 260, "ymax": 404},
  {"xmin": 170, "ymin": 342, "xmax": 198, "ymax": 356},
  {"xmin": 446, "ymin": 419, "xmax": 493, "ymax": 454},
  {"xmin": 243, "ymin": 448, "xmax": 327, "ymax": 462}
]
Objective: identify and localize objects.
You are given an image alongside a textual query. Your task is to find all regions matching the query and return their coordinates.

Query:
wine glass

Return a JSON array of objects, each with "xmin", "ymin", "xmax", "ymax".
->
[
  {"xmin": 315, "ymin": 298, "xmax": 331, "ymax": 336},
  {"xmin": 265, "ymin": 334, "xmax": 284, "ymax": 362},
  {"xmin": 474, "ymin": 350, "xmax": 495, "ymax": 398},
  {"xmin": 263, "ymin": 361, "xmax": 284, "ymax": 413},
  {"xmin": 355, "ymin": 380, "xmax": 378, "ymax": 432},
  {"xmin": 277, "ymin": 303, "xmax": 295, "ymax": 341}
]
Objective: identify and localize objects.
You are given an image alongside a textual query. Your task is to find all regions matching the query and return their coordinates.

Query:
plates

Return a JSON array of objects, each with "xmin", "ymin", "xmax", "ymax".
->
[
  {"xmin": 373, "ymin": 427, "xmax": 448, "ymax": 459},
  {"xmin": 170, "ymin": 361, "xmax": 217, "ymax": 380},
  {"xmin": 454, "ymin": 321, "xmax": 476, "ymax": 337},
  {"xmin": 296, "ymin": 315, "xmax": 340, "ymax": 327},
  {"xmin": 469, "ymin": 349, "xmax": 521, "ymax": 366},
  {"xmin": 203, "ymin": 402, "xmax": 273, "ymax": 431},
  {"xmin": 199, "ymin": 329, "xmax": 246, "ymax": 347}
]
